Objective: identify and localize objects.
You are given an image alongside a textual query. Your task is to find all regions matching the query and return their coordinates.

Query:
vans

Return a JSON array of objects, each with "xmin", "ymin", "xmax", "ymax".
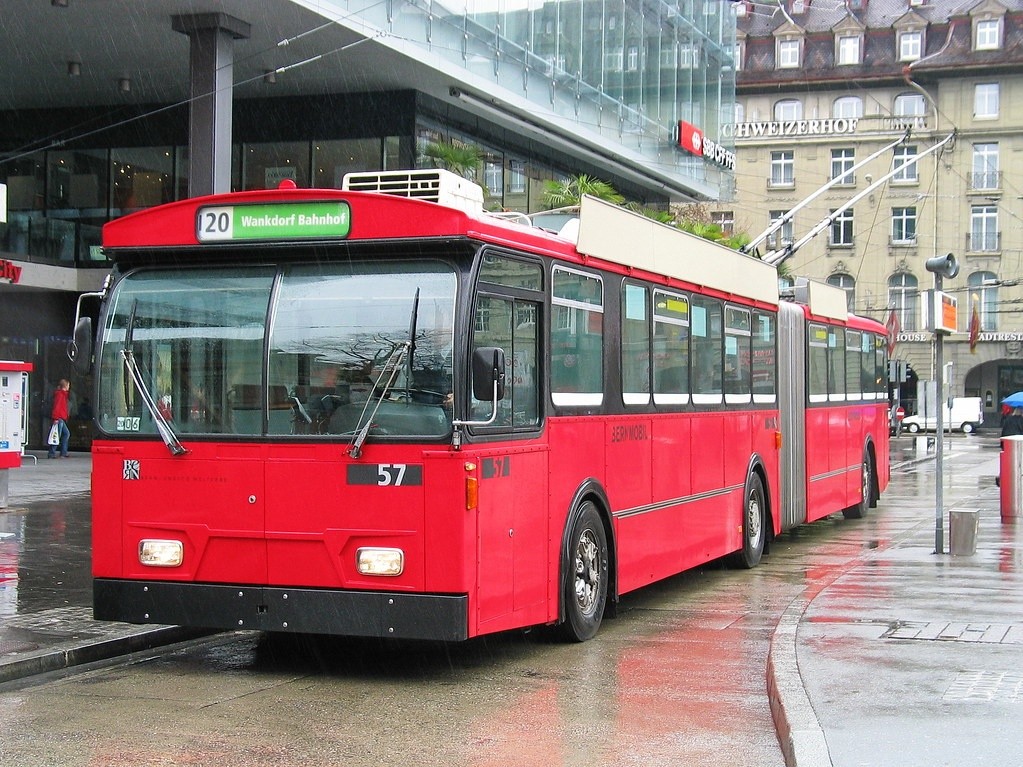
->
[{"xmin": 901, "ymin": 398, "xmax": 984, "ymax": 434}]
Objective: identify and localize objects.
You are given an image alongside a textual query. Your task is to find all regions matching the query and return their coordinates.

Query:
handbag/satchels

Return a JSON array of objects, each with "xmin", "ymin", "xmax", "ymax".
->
[{"xmin": 48, "ymin": 424, "xmax": 59, "ymax": 445}]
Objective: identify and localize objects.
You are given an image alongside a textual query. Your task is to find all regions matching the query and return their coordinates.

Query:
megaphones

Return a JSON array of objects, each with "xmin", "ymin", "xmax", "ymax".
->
[{"xmin": 926, "ymin": 253, "xmax": 960, "ymax": 279}]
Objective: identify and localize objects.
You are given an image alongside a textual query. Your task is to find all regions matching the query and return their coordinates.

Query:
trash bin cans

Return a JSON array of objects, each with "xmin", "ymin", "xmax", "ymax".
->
[{"xmin": 999, "ymin": 434, "xmax": 1023, "ymax": 526}]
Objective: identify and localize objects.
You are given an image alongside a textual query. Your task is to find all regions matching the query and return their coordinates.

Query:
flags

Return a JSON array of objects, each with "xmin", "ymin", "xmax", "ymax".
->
[
  {"xmin": 885, "ymin": 310, "xmax": 900, "ymax": 359},
  {"xmin": 970, "ymin": 306, "xmax": 980, "ymax": 354}
]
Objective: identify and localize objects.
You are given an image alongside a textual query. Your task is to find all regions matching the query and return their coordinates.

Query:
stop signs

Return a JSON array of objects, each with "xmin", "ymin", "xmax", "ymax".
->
[{"xmin": 896, "ymin": 407, "xmax": 905, "ymax": 420}]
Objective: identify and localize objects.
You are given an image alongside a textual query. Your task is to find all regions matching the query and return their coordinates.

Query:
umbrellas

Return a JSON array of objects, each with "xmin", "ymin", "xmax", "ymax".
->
[{"xmin": 999, "ymin": 392, "xmax": 1023, "ymax": 407}]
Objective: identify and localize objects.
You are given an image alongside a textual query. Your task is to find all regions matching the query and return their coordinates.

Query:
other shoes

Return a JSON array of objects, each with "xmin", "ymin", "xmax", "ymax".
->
[
  {"xmin": 48, "ymin": 455, "xmax": 56, "ymax": 459},
  {"xmin": 60, "ymin": 455, "xmax": 69, "ymax": 457}
]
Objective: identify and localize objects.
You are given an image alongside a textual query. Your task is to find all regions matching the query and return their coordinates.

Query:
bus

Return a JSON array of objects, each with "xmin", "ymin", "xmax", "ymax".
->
[{"xmin": 68, "ymin": 163, "xmax": 890, "ymax": 645}]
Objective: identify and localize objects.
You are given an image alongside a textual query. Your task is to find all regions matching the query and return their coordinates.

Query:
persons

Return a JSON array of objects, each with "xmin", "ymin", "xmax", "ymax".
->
[
  {"xmin": 389, "ymin": 298, "xmax": 480, "ymax": 409},
  {"xmin": 995, "ymin": 406, "xmax": 1023, "ymax": 487},
  {"xmin": 47, "ymin": 379, "xmax": 71, "ymax": 459},
  {"xmin": 1000, "ymin": 396, "xmax": 1011, "ymax": 427}
]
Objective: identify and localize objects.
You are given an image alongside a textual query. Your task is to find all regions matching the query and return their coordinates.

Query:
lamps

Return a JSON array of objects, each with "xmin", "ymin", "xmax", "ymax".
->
[
  {"xmin": 264, "ymin": 70, "xmax": 277, "ymax": 84},
  {"xmin": 449, "ymin": 86, "xmax": 701, "ymax": 204},
  {"xmin": 66, "ymin": 63, "xmax": 81, "ymax": 76},
  {"xmin": 117, "ymin": 78, "xmax": 130, "ymax": 93}
]
